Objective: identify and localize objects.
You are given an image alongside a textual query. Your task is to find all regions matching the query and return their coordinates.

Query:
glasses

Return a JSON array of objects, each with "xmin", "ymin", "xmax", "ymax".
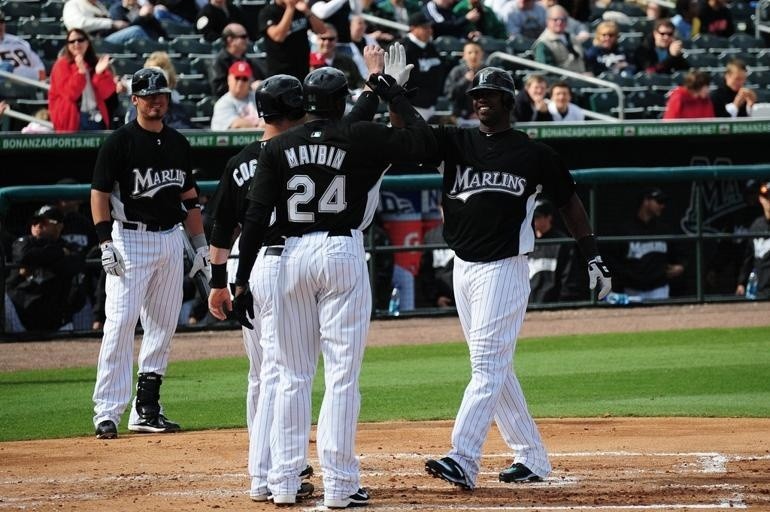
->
[
  {"xmin": 319, "ymin": 36, "xmax": 338, "ymax": 42},
  {"xmin": 0, "ymin": 19, "xmax": 6, "ymax": 24},
  {"xmin": 231, "ymin": 34, "xmax": 249, "ymax": 40},
  {"xmin": 656, "ymin": 28, "xmax": 674, "ymax": 37},
  {"xmin": 68, "ymin": 36, "xmax": 87, "ymax": 45},
  {"xmin": 235, "ymin": 76, "xmax": 248, "ymax": 82}
]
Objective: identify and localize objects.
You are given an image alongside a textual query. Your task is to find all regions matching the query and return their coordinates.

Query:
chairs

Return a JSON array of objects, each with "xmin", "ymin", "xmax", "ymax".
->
[
  {"xmin": 1, "ymin": 0, "xmax": 287, "ymax": 131},
  {"xmin": 433, "ymin": 0, "xmax": 770, "ymax": 120}
]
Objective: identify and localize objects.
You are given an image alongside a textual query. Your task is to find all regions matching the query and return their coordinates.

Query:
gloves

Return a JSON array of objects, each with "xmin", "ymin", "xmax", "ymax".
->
[
  {"xmin": 576, "ymin": 232, "xmax": 618, "ymax": 302},
  {"xmin": 188, "ymin": 244, "xmax": 212, "ymax": 283},
  {"xmin": 363, "ymin": 69, "xmax": 409, "ymax": 114},
  {"xmin": 380, "ymin": 40, "xmax": 418, "ymax": 86},
  {"xmin": 99, "ymin": 242, "xmax": 126, "ymax": 277},
  {"xmin": 229, "ymin": 280, "xmax": 256, "ymax": 330}
]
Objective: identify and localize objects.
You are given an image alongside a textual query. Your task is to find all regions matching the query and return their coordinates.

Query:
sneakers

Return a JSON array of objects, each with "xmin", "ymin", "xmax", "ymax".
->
[
  {"xmin": 127, "ymin": 406, "xmax": 182, "ymax": 434},
  {"xmin": 322, "ymin": 486, "xmax": 371, "ymax": 509},
  {"xmin": 424, "ymin": 455, "xmax": 474, "ymax": 489},
  {"xmin": 498, "ymin": 462, "xmax": 542, "ymax": 483},
  {"xmin": 270, "ymin": 481, "xmax": 314, "ymax": 508},
  {"xmin": 95, "ymin": 418, "xmax": 118, "ymax": 440}
]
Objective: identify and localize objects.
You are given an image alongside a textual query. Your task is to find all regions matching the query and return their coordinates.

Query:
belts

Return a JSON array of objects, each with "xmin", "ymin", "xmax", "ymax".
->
[
  {"xmin": 118, "ymin": 219, "xmax": 183, "ymax": 233},
  {"xmin": 283, "ymin": 228, "xmax": 353, "ymax": 238},
  {"xmin": 256, "ymin": 245, "xmax": 285, "ymax": 257}
]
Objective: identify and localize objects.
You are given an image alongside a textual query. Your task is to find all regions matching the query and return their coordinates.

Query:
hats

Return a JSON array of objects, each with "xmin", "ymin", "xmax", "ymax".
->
[
  {"xmin": 405, "ymin": 10, "xmax": 436, "ymax": 28},
  {"xmin": 533, "ymin": 198, "xmax": 558, "ymax": 218},
  {"xmin": 226, "ymin": 59, "xmax": 252, "ymax": 80},
  {"xmin": 639, "ymin": 185, "xmax": 672, "ymax": 203},
  {"xmin": 33, "ymin": 202, "xmax": 66, "ymax": 225},
  {"xmin": 308, "ymin": 51, "xmax": 326, "ymax": 67}
]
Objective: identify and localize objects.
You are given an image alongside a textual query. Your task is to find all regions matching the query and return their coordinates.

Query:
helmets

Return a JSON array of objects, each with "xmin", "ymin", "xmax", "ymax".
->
[
  {"xmin": 253, "ymin": 73, "xmax": 307, "ymax": 120},
  {"xmin": 301, "ymin": 64, "xmax": 356, "ymax": 111},
  {"xmin": 131, "ymin": 66, "xmax": 173, "ymax": 97},
  {"xmin": 462, "ymin": 64, "xmax": 516, "ymax": 102}
]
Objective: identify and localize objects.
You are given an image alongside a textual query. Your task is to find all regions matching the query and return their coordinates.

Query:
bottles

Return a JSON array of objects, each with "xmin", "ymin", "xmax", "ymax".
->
[
  {"xmin": 745, "ymin": 268, "xmax": 759, "ymax": 299},
  {"xmin": 604, "ymin": 293, "xmax": 629, "ymax": 305},
  {"xmin": 387, "ymin": 284, "xmax": 401, "ymax": 317}
]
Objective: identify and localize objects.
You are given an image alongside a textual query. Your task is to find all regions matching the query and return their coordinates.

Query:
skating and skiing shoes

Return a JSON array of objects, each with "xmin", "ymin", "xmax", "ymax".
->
[{"xmin": 248, "ymin": 462, "xmax": 315, "ymax": 502}]
